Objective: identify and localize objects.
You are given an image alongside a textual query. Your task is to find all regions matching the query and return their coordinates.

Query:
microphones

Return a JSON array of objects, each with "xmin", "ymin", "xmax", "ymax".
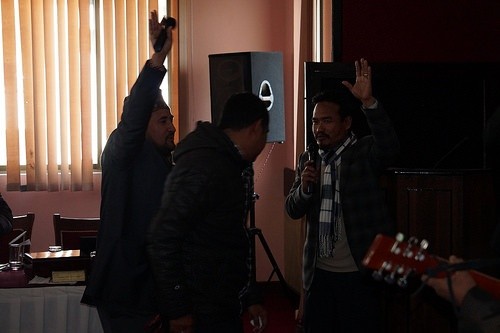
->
[{"xmin": 306, "ymin": 142, "xmax": 319, "ymax": 193}]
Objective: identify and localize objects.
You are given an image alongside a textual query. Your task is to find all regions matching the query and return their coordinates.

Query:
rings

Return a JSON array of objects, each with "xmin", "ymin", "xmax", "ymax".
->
[{"xmin": 363, "ymin": 72, "xmax": 368, "ymax": 76}]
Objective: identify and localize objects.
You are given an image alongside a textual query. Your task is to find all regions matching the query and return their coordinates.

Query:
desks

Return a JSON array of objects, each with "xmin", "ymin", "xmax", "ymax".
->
[{"xmin": 0, "ymin": 272, "xmax": 104, "ymax": 333}]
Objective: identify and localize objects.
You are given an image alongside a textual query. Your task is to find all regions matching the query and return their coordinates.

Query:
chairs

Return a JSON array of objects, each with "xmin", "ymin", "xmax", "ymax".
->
[
  {"xmin": 53, "ymin": 213, "xmax": 100, "ymax": 257},
  {"xmin": 9, "ymin": 212, "xmax": 35, "ymax": 261}
]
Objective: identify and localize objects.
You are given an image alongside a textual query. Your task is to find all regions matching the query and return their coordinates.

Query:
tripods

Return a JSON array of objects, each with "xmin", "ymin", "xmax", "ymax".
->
[{"xmin": 246, "ymin": 183, "xmax": 297, "ymax": 310}]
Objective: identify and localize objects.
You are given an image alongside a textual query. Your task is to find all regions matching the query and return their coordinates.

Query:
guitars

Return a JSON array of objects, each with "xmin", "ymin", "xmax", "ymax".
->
[{"xmin": 364, "ymin": 235, "xmax": 500, "ymax": 302}]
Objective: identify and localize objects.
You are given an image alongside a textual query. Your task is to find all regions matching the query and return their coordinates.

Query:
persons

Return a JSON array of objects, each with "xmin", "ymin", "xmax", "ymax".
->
[
  {"xmin": 80, "ymin": 10, "xmax": 214, "ymax": 333},
  {"xmin": 146, "ymin": 93, "xmax": 269, "ymax": 333},
  {"xmin": 420, "ymin": 255, "xmax": 500, "ymax": 333},
  {"xmin": 284, "ymin": 58, "xmax": 400, "ymax": 333},
  {"xmin": 0, "ymin": 193, "xmax": 13, "ymax": 235}
]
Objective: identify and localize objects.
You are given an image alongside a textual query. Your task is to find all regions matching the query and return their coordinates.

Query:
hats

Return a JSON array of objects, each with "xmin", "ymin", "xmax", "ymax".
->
[{"xmin": 123, "ymin": 89, "xmax": 170, "ymax": 112}]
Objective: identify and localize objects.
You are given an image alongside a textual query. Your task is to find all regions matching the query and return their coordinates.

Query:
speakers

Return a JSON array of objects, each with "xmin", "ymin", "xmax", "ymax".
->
[{"xmin": 207, "ymin": 51, "xmax": 286, "ymax": 144}]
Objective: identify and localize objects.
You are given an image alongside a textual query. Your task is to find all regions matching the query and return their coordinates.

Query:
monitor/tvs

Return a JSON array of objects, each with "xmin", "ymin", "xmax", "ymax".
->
[{"xmin": 304, "ymin": 61, "xmax": 500, "ymax": 176}]
